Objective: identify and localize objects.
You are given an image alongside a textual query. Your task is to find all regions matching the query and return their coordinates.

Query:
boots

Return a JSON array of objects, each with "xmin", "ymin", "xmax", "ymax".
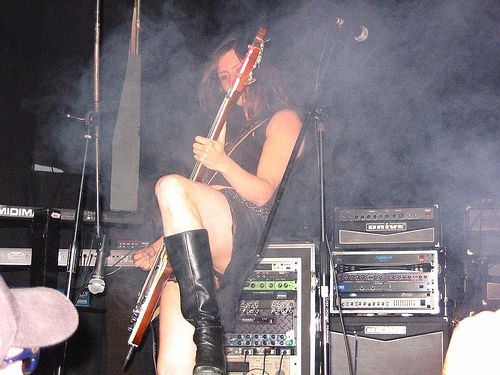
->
[{"xmin": 163, "ymin": 230, "xmax": 226, "ymax": 375}]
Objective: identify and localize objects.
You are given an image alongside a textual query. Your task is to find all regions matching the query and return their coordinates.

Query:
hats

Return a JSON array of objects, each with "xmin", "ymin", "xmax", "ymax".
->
[{"xmin": 0, "ymin": 273, "xmax": 79, "ymax": 365}]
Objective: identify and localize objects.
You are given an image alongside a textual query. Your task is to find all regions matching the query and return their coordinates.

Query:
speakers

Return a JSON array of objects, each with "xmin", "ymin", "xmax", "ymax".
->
[
  {"xmin": 0, "ymin": 223, "xmax": 159, "ymax": 375},
  {"xmin": 329, "ymin": 314, "xmax": 449, "ymax": 375}
]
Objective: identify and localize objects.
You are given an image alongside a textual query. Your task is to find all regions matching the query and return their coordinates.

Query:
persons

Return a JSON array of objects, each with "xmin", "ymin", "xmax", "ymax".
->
[
  {"xmin": 0, "ymin": 271, "xmax": 80, "ymax": 374},
  {"xmin": 132, "ymin": 29, "xmax": 310, "ymax": 375}
]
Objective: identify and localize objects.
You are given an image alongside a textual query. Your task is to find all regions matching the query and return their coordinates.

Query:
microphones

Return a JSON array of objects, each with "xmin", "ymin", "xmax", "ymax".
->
[
  {"xmin": 87, "ymin": 233, "xmax": 108, "ymax": 295},
  {"xmin": 332, "ymin": 17, "xmax": 369, "ymax": 42}
]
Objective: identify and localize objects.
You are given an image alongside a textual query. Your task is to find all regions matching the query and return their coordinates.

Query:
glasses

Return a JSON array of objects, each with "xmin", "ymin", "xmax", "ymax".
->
[{"xmin": 1, "ymin": 348, "xmax": 40, "ymax": 375}]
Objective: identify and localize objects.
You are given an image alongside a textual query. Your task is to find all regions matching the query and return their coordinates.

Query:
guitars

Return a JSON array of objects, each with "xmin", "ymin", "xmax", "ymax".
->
[{"xmin": 126, "ymin": 27, "xmax": 270, "ymax": 348}]
